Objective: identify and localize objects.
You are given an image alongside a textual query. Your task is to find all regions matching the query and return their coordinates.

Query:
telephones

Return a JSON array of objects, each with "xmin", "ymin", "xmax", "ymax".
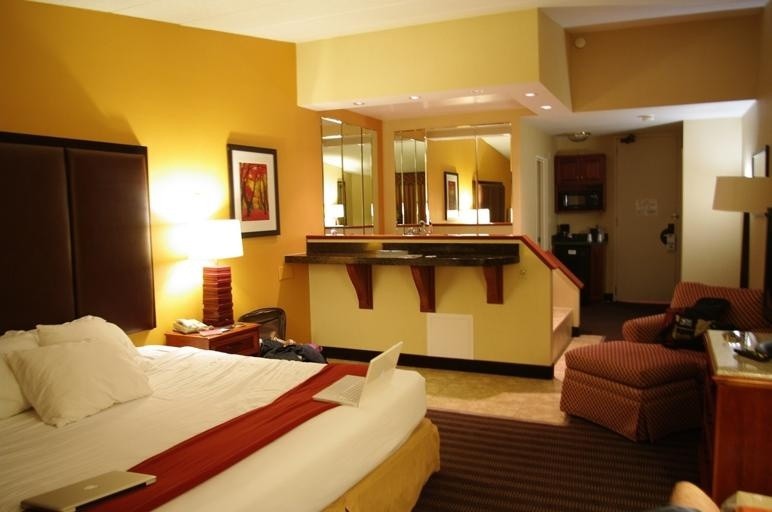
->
[{"xmin": 173, "ymin": 318, "xmax": 209, "ymax": 334}]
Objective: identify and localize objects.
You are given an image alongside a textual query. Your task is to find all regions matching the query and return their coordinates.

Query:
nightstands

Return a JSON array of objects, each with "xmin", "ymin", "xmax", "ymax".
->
[{"xmin": 164, "ymin": 321, "xmax": 262, "ymax": 357}]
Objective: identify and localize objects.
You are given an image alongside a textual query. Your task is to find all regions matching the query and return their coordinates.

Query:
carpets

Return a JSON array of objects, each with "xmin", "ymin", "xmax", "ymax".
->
[{"xmin": 410, "ymin": 407, "xmax": 702, "ymax": 511}]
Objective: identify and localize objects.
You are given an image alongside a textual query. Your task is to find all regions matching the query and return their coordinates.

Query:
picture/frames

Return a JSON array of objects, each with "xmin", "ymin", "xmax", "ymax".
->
[
  {"xmin": 226, "ymin": 143, "xmax": 280, "ymax": 238},
  {"xmin": 392, "ymin": 122, "xmax": 514, "ymax": 226},
  {"xmin": 444, "ymin": 171, "xmax": 459, "ymax": 221},
  {"xmin": 750, "ymin": 143, "xmax": 769, "ymax": 176}
]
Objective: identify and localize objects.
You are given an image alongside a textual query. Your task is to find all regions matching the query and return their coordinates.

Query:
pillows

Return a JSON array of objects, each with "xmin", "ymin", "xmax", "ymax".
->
[
  {"xmin": 0, "ymin": 315, "xmax": 153, "ymax": 430},
  {"xmin": 662, "ymin": 312, "xmax": 720, "ymax": 352}
]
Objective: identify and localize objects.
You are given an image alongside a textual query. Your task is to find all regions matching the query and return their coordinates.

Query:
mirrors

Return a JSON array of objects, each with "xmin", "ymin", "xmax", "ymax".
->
[
  {"xmin": 394, "ymin": 128, "xmax": 430, "ymax": 225},
  {"xmin": 319, "ymin": 115, "xmax": 374, "ymax": 236}
]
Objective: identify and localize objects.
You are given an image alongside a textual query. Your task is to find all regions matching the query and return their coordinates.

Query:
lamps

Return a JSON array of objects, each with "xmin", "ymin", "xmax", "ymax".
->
[
  {"xmin": 189, "ymin": 219, "xmax": 246, "ymax": 327},
  {"xmin": 711, "ymin": 175, "xmax": 772, "ymax": 287}
]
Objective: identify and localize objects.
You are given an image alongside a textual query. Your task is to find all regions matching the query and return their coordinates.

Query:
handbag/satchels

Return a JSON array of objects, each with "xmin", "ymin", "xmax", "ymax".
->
[{"xmin": 658, "ymin": 295, "xmax": 732, "ymax": 352}]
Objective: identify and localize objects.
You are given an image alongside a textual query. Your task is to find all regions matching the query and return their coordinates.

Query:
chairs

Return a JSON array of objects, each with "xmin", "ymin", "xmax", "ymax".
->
[{"xmin": 622, "ymin": 280, "xmax": 766, "ymax": 409}]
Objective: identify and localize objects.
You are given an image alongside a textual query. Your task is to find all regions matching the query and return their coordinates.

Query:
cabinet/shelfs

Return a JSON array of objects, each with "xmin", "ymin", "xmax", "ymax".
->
[
  {"xmin": 554, "ymin": 154, "xmax": 606, "ymax": 212},
  {"xmin": 552, "ymin": 232, "xmax": 609, "ymax": 305},
  {"xmin": 700, "ymin": 328, "xmax": 771, "ymax": 507}
]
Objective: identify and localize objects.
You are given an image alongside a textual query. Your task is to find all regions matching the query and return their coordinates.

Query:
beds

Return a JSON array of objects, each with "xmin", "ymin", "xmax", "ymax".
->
[{"xmin": 0, "ymin": 129, "xmax": 444, "ymax": 511}]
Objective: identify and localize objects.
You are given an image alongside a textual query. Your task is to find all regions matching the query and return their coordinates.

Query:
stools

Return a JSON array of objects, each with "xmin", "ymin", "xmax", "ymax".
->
[{"xmin": 559, "ymin": 339, "xmax": 706, "ymax": 444}]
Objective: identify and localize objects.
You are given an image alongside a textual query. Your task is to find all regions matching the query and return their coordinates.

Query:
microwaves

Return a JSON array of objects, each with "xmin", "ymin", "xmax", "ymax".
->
[{"xmin": 559, "ymin": 190, "xmax": 603, "ymax": 211}]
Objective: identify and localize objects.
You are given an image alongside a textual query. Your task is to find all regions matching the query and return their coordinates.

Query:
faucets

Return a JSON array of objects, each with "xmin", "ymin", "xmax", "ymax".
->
[{"xmin": 418, "ymin": 220, "xmax": 426, "ymax": 230}]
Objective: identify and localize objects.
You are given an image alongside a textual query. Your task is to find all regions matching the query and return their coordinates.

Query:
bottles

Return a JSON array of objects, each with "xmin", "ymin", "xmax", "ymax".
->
[
  {"xmin": 587, "ymin": 233, "xmax": 593, "ymax": 242},
  {"xmin": 598, "ymin": 233, "xmax": 602, "ymax": 243}
]
